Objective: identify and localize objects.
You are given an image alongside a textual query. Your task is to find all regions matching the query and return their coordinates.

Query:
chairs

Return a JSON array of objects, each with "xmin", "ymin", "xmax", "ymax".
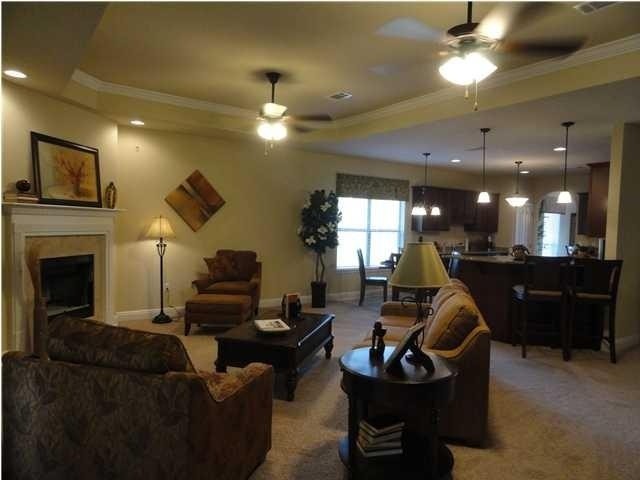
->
[
  {"xmin": 568, "ymin": 257, "xmax": 616, "ymax": 364},
  {"xmin": 392, "ymin": 254, "xmax": 401, "ymax": 269},
  {"xmin": 511, "ymin": 253, "xmax": 568, "ymax": 363},
  {"xmin": 357, "ymin": 249, "xmax": 388, "ymax": 305}
]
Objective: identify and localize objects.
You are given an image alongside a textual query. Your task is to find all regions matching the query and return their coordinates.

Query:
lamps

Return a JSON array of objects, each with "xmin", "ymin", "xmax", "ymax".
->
[
  {"xmin": 554, "ymin": 121, "xmax": 574, "ymax": 204},
  {"xmin": 410, "ymin": 154, "xmax": 441, "ymax": 216},
  {"xmin": 438, "ymin": 9, "xmax": 497, "ymax": 110},
  {"xmin": 388, "ymin": 243, "xmax": 454, "ymax": 367},
  {"xmin": 256, "ymin": 72, "xmax": 290, "ymax": 157},
  {"xmin": 145, "ymin": 217, "xmax": 175, "ymax": 323},
  {"xmin": 505, "ymin": 161, "xmax": 530, "ymax": 210},
  {"xmin": 476, "ymin": 128, "xmax": 492, "ymax": 204}
]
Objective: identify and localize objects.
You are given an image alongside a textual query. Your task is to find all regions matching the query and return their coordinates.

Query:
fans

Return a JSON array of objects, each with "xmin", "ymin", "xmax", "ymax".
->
[{"xmin": 377, "ymin": 1, "xmax": 589, "ymax": 110}]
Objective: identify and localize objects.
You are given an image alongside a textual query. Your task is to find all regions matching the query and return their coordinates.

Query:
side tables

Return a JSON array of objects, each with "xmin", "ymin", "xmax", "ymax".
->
[{"xmin": 338, "ymin": 346, "xmax": 459, "ymax": 480}]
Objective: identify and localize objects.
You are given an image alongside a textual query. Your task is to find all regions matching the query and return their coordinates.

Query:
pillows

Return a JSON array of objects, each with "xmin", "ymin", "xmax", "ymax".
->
[
  {"xmin": 42, "ymin": 317, "xmax": 196, "ymax": 374},
  {"xmin": 420, "ymin": 279, "xmax": 470, "ymax": 327},
  {"xmin": 203, "ymin": 257, "xmax": 225, "ymax": 283},
  {"xmin": 214, "ymin": 255, "xmax": 242, "ymax": 281},
  {"xmin": 424, "ymin": 292, "xmax": 476, "ymax": 347}
]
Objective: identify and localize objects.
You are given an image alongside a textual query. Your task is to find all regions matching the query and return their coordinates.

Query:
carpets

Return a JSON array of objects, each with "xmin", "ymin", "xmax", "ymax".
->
[{"xmin": 118, "ymin": 319, "xmax": 349, "ymax": 480}]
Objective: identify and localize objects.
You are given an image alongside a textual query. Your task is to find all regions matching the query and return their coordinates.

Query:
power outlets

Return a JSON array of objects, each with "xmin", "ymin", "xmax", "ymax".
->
[{"xmin": 164, "ymin": 283, "xmax": 172, "ymax": 295}]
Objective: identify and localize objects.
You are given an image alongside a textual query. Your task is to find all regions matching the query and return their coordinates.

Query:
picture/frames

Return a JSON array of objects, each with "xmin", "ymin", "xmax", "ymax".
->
[
  {"xmin": 382, "ymin": 322, "xmax": 437, "ymax": 374},
  {"xmin": 31, "ymin": 131, "xmax": 102, "ymax": 207},
  {"xmin": 163, "ymin": 169, "xmax": 226, "ymax": 234}
]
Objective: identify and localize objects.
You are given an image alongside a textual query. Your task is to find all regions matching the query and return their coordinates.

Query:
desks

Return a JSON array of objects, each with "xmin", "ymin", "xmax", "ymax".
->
[{"xmin": 446, "ymin": 251, "xmax": 612, "ymax": 343}]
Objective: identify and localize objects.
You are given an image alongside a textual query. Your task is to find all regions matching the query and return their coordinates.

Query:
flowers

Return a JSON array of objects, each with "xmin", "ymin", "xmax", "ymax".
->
[{"xmin": 296, "ymin": 189, "xmax": 342, "ymax": 282}]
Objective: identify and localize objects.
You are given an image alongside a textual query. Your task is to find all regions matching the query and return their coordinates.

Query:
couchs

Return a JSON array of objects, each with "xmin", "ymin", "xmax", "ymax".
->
[
  {"xmin": 184, "ymin": 249, "xmax": 262, "ymax": 335},
  {"xmin": 340, "ymin": 279, "xmax": 489, "ymax": 445},
  {"xmin": 2, "ymin": 313, "xmax": 273, "ymax": 479}
]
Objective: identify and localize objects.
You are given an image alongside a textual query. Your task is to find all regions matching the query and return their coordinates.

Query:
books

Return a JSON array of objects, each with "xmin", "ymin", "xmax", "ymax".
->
[
  {"xmin": 356, "ymin": 412, "xmax": 404, "ymax": 459},
  {"xmin": 256, "ymin": 318, "xmax": 289, "ymax": 333}
]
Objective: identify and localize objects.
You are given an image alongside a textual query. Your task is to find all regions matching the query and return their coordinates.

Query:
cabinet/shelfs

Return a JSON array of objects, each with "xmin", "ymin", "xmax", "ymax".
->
[
  {"xmin": 412, "ymin": 186, "xmax": 500, "ymax": 233},
  {"xmin": 434, "ymin": 245, "xmax": 509, "ymax": 272},
  {"xmin": 586, "ymin": 162, "xmax": 612, "ymax": 238},
  {"xmin": 3, "ymin": 203, "xmax": 125, "ymax": 353}
]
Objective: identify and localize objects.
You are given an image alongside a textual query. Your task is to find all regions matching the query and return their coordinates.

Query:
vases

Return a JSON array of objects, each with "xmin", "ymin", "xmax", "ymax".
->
[{"xmin": 310, "ymin": 281, "xmax": 327, "ymax": 308}]
